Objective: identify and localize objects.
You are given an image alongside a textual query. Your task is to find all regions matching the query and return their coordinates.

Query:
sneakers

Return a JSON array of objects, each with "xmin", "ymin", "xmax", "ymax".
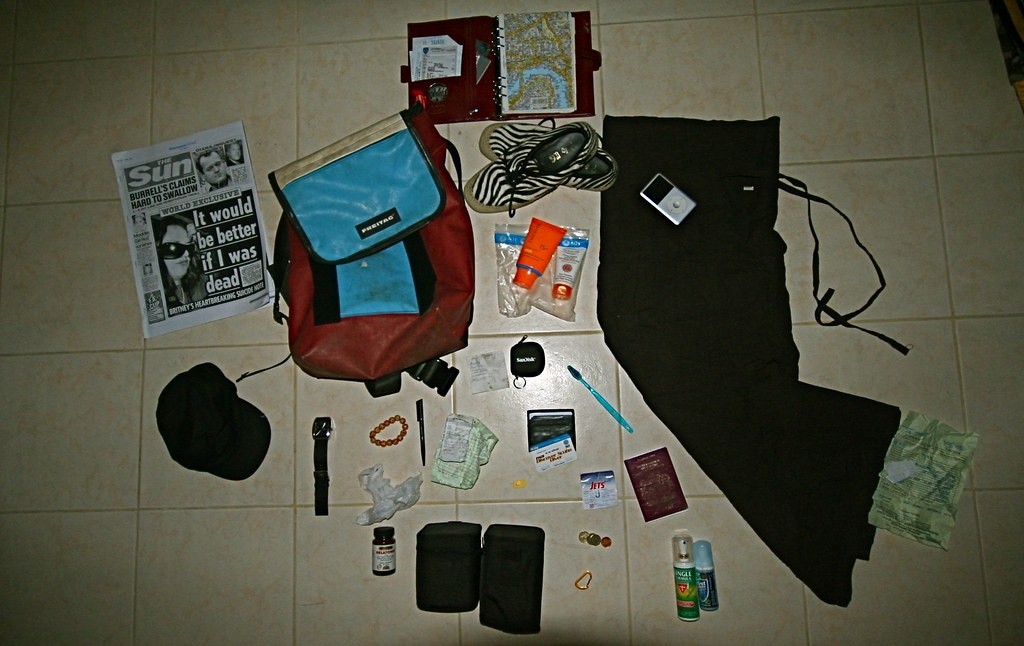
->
[{"xmin": 465, "ymin": 117, "xmax": 618, "ymax": 218}]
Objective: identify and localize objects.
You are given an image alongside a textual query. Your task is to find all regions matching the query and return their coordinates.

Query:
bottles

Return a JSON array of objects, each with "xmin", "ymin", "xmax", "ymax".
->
[
  {"xmin": 672, "ymin": 535, "xmax": 700, "ymax": 622},
  {"xmin": 693, "ymin": 540, "xmax": 719, "ymax": 611},
  {"xmin": 372, "ymin": 526, "xmax": 396, "ymax": 576}
]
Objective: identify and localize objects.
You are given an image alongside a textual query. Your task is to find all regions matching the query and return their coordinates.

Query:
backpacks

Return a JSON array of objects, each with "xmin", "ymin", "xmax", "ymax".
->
[{"xmin": 269, "ymin": 101, "xmax": 476, "ymax": 398}]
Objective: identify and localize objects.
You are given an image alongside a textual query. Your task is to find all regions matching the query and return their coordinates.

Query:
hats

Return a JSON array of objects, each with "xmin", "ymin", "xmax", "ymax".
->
[{"xmin": 156, "ymin": 362, "xmax": 272, "ymax": 480}]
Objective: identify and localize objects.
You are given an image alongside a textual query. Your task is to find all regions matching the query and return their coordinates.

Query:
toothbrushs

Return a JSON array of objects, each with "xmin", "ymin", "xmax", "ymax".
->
[{"xmin": 565, "ymin": 364, "xmax": 635, "ymax": 433}]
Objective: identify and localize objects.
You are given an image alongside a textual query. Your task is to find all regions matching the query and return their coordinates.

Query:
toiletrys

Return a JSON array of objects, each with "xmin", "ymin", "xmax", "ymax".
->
[{"xmin": 511, "ymin": 216, "xmax": 590, "ymax": 300}]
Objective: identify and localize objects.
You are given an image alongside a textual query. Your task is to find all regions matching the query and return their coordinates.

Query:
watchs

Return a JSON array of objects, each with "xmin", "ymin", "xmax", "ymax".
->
[{"xmin": 311, "ymin": 417, "xmax": 332, "ymax": 515}]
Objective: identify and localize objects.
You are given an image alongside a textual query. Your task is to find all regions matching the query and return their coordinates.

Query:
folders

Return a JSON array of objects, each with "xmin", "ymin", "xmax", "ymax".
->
[{"xmin": 400, "ymin": 11, "xmax": 602, "ymax": 125}]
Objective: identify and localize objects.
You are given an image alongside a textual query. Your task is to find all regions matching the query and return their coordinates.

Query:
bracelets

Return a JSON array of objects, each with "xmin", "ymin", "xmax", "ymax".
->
[{"xmin": 370, "ymin": 415, "xmax": 408, "ymax": 448}]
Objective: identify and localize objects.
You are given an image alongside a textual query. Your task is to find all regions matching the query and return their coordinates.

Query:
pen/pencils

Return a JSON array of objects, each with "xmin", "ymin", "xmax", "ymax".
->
[{"xmin": 416, "ymin": 398, "xmax": 426, "ymax": 466}]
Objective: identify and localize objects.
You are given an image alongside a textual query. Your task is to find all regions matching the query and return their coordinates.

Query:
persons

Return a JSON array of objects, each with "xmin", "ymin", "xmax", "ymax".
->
[
  {"xmin": 227, "ymin": 142, "xmax": 241, "ymax": 166},
  {"xmin": 156, "ymin": 215, "xmax": 209, "ymax": 311},
  {"xmin": 194, "ymin": 149, "xmax": 232, "ymax": 191}
]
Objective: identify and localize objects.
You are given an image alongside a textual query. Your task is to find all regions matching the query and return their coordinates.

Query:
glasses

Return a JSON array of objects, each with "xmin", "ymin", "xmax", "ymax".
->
[{"xmin": 161, "ymin": 242, "xmax": 195, "ymax": 259}]
